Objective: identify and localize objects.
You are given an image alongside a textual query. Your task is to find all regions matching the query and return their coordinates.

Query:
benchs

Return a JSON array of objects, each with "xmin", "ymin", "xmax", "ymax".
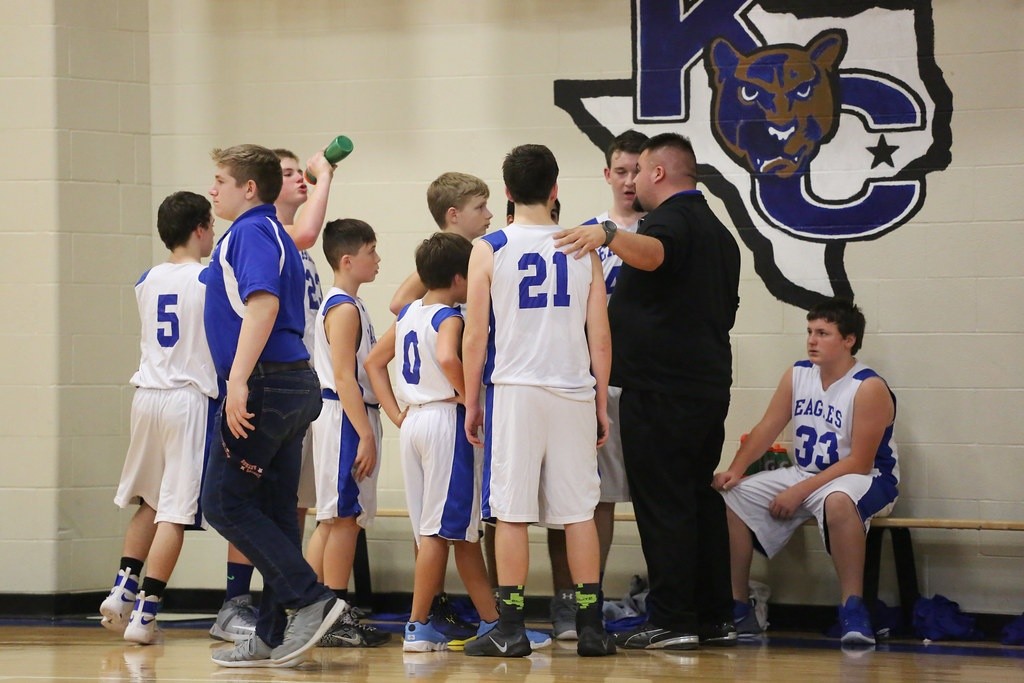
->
[{"xmin": 297, "ymin": 507, "xmax": 1024, "ymax": 625}]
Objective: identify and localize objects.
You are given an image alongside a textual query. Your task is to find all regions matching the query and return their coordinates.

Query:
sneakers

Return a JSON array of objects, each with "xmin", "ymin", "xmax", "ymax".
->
[
  {"xmin": 477, "ymin": 619, "xmax": 552, "ymax": 649},
  {"xmin": 550, "ymin": 590, "xmax": 579, "ymax": 640},
  {"xmin": 101, "ymin": 567, "xmax": 165, "ymax": 645},
  {"xmin": 209, "ymin": 594, "xmax": 259, "ymax": 645},
  {"xmin": 211, "ymin": 633, "xmax": 274, "ymax": 668},
  {"xmin": 838, "ymin": 595, "xmax": 875, "ymax": 645},
  {"xmin": 464, "ymin": 626, "xmax": 532, "ymax": 657},
  {"xmin": 271, "ymin": 594, "xmax": 346, "ymax": 667},
  {"xmin": 616, "ymin": 615, "xmax": 737, "ymax": 650},
  {"xmin": 733, "ymin": 598, "xmax": 764, "ymax": 638},
  {"xmin": 315, "ymin": 601, "xmax": 392, "ymax": 647},
  {"xmin": 402, "ymin": 618, "xmax": 448, "ymax": 652},
  {"xmin": 577, "ymin": 625, "xmax": 617, "ymax": 657}
]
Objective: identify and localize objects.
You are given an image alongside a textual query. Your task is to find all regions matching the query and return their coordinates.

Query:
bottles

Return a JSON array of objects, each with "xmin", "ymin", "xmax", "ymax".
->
[
  {"xmin": 737, "ymin": 433, "xmax": 792, "ymax": 479},
  {"xmin": 303, "ymin": 134, "xmax": 354, "ymax": 186}
]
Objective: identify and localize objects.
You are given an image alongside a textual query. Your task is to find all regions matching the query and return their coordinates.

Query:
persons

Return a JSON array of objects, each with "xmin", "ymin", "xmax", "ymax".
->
[
  {"xmin": 199, "ymin": 145, "xmax": 347, "ymax": 667},
  {"xmin": 552, "ymin": 132, "xmax": 738, "ymax": 648},
  {"xmin": 389, "ymin": 171, "xmax": 477, "ymax": 643},
  {"xmin": 579, "ymin": 130, "xmax": 651, "ymax": 628},
  {"xmin": 483, "ymin": 195, "xmax": 579, "ymax": 642},
  {"xmin": 365, "ymin": 232, "xmax": 552, "ymax": 652},
  {"xmin": 306, "ymin": 218, "xmax": 390, "ymax": 647},
  {"xmin": 207, "ymin": 149, "xmax": 336, "ymax": 639},
  {"xmin": 462, "ymin": 143, "xmax": 616, "ymax": 656},
  {"xmin": 97, "ymin": 190, "xmax": 215, "ymax": 644},
  {"xmin": 712, "ymin": 301, "xmax": 901, "ymax": 645}
]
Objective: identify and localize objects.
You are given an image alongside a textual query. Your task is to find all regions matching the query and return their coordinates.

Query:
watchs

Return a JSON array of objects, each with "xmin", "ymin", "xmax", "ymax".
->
[{"xmin": 600, "ymin": 220, "xmax": 617, "ymax": 247}]
